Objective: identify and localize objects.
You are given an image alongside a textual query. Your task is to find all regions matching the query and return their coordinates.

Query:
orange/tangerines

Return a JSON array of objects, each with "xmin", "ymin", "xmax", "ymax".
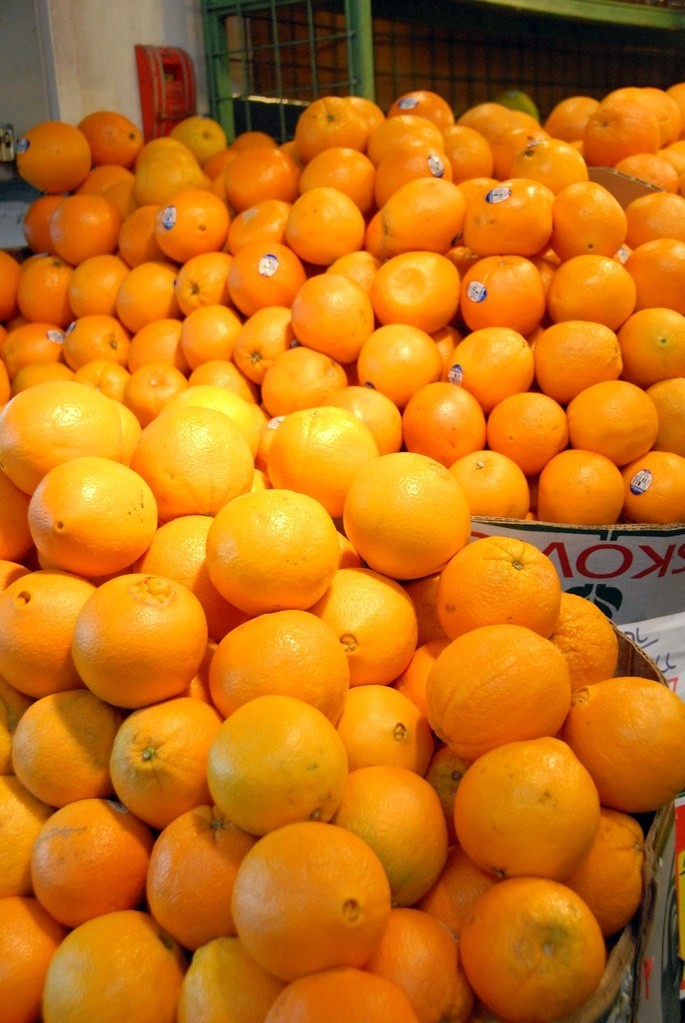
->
[
  {"xmin": 0, "ymin": 83, "xmax": 685, "ymax": 528},
  {"xmin": 0, "ymin": 452, "xmax": 685, "ymax": 1023}
]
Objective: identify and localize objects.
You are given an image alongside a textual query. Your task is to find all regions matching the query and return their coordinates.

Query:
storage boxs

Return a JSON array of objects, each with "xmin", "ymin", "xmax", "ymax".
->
[{"xmin": 470, "ymin": 516, "xmax": 685, "ymax": 1023}]
